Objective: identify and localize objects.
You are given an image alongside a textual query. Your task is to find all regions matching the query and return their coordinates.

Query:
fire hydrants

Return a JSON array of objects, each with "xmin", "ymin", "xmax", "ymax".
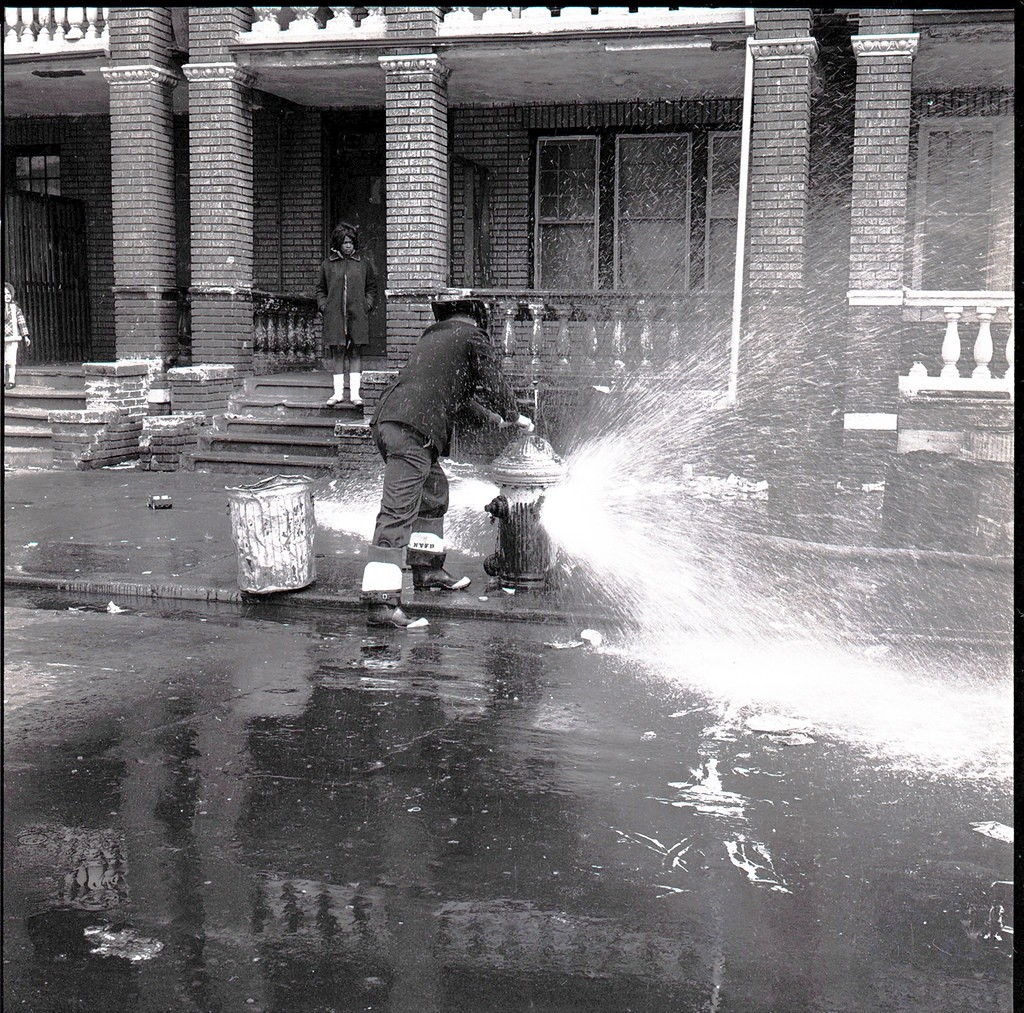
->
[{"xmin": 482, "ymin": 427, "xmax": 566, "ymax": 596}]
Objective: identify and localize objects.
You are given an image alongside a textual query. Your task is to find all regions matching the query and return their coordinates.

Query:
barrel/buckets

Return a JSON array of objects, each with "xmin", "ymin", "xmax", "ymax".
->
[{"xmin": 223, "ymin": 473, "xmax": 317, "ymax": 595}]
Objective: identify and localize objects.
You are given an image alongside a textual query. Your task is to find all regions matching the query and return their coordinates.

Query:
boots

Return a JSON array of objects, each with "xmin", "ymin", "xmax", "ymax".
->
[
  {"xmin": 349, "ymin": 372, "xmax": 364, "ymax": 406},
  {"xmin": 325, "ymin": 374, "xmax": 344, "ymax": 406},
  {"xmin": 357, "ymin": 546, "xmax": 428, "ymax": 630},
  {"xmin": 406, "ymin": 515, "xmax": 470, "ymax": 589}
]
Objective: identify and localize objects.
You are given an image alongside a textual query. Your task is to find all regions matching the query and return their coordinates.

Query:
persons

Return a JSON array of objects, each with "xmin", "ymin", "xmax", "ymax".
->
[
  {"xmin": 358, "ymin": 294, "xmax": 536, "ymax": 631},
  {"xmin": 316, "ymin": 222, "xmax": 380, "ymax": 408},
  {"xmin": 3, "ymin": 282, "xmax": 31, "ymax": 389}
]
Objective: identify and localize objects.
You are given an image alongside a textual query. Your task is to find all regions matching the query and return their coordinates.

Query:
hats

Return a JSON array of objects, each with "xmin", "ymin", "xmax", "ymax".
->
[{"xmin": 432, "ymin": 299, "xmax": 488, "ymax": 331}]
